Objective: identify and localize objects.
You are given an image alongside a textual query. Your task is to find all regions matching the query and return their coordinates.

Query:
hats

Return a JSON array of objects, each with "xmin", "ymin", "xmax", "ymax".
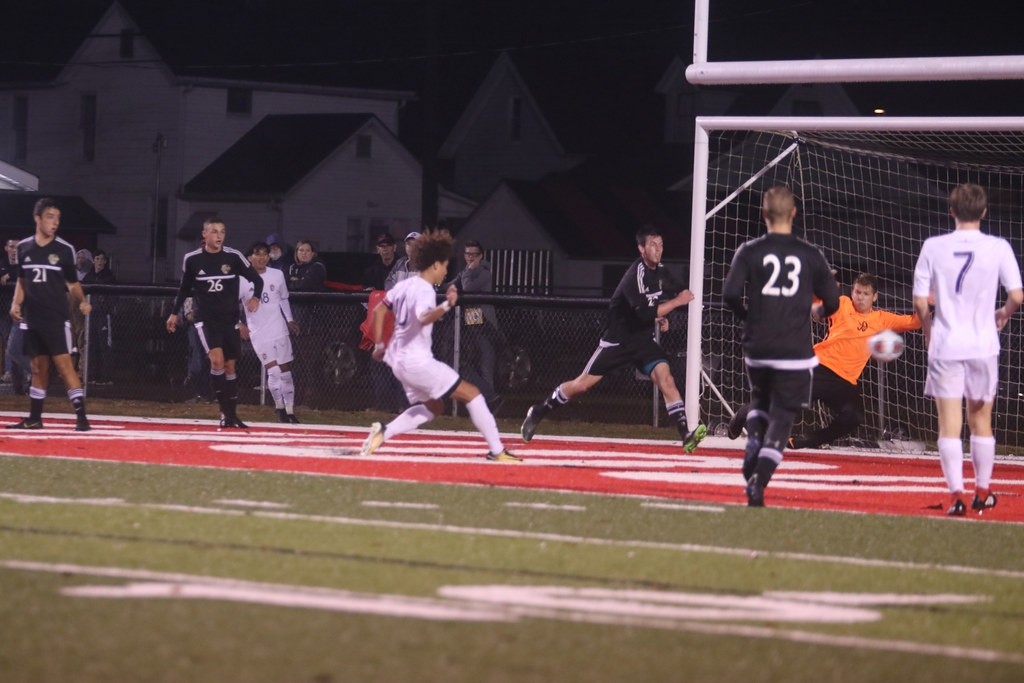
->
[
  {"xmin": 405, "ymin": 231, "xmax": 422, "ymax": 242},
  {"xmin": 376, "ymin": 233, "xmax": 394, "ymax": 246}
]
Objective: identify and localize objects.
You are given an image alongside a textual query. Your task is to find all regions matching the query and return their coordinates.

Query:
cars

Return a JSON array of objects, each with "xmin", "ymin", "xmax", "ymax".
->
[{"xmin": 289, "ymin": 287, "xmax": 733, "ymax": 414}]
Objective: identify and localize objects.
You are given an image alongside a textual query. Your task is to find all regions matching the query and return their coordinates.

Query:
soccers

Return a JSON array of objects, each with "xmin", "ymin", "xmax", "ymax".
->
[{"xmin": 867, "ymin": 329, "xmax": 904, "ymax": 362}]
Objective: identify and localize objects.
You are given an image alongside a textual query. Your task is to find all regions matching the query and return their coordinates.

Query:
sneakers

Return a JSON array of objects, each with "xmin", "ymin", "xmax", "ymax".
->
[
  {"xmin": 360, "ymin": 421, "xmax": 386, "ymax": 457},
  {"xmin": 276, "ymin": 409, "xmax": 289, "ymax": 424},
  {"xmin": 745, "ymin": 474, "xmax": 764, "ymax": 507},
  {"xmin": 785, "ymin": 436, "xmax": 805, "ymax": 450},
  {"xmin": 219, "ymin": 415, "xmax": 234, "ymax": 430},
  {"xmin": 232, "ymin": 416, "xmax": 248, "ymax": 429},
  {"xmin": 75, "ymin": 417, "xmax": 90, "ymax": 431},
  {"xmin": 6, "ymin": 417, "xmax": 44, "ymax": 429},
  {"xmin": 728, "ymin": 406, "xmax": 746, "ymax": 439},
  {"xmin": 287, "ymin": 414, "xmax": 298, "ymax": 424},
  {"xmin": 683, "ymin": 424, "xmax": 706, "ymax": 454},
  {"xmin": 947, "ymin": 491, "xmax": 967, "ymax": 516},
  {"xmin": 521, "ymin": 405, "xmax": 539, "ymax": 443},
  {"xmin": 743, "ymin": 436, "xmax": 760, "ymax": 481},
  {"xmin": 486, "ymin": 447, "xmax": 524, "ymax": 461},
  {"xmin": 971, "ymin": 490, "xmax": 997, "ymax": 510}
]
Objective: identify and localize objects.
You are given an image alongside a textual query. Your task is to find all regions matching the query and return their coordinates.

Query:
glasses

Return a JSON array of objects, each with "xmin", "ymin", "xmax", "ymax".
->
[{"xmin": 465, "ymin": 251, "xmax": 482, "ymax": 257}]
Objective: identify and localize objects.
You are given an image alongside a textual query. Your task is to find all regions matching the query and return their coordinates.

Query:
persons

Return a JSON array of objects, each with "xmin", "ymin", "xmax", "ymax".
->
[
  {"xmin": 288, "ymin": 240, "xmax": 325, "ymax": 291},
  {"xmin": 238, "ymin": 241, "xmax": 300, "ymax": 424},
  {"xmin": 364, "ymin": 233, "xmax": 401, "ymax": 291},
  {"xmin": 384, "ymin": 231, "xmax": 422, "ymax": 292},
  {"xmin": 5, "ymin": 198, "xmax": 90, "ymax": 431},
  {"xmin": 438, "ymin": 241, "xmax": 498, "ymax": 398},
  {"xmin": 166, "ymin": 217, "xmax": 264, "ymax": 428},
  {"xmin": 722, "ymin": 187, "xmax": 840, "ymax": 507},
  {"xmin": 912, "ymin": 184, "xmax": 1024, "ymax": 515},
  {"xmin": 727, "ymin": 274, "xmax": 923, "ymax": 450},
  {"xmin": 521, "ymin": 227, "xmax": 708, "ymax": 453},
  {"xmin": 81, "ymin": 251, "xmax": 120, "ymax": 386},
  {"xmin": 183, "ymin": 296, "xmax": 211, "ymax": 398},
  {"xmin": 360, "ymin": 229, "xmax": 524, "ymax": 462},
  {"xmin": 265, "ymin": 232, "xmax": 291, "ymax": 287},
  {"xmin": 0, "ymin": 234, "xmax": 32, "ymax": 396}
]
